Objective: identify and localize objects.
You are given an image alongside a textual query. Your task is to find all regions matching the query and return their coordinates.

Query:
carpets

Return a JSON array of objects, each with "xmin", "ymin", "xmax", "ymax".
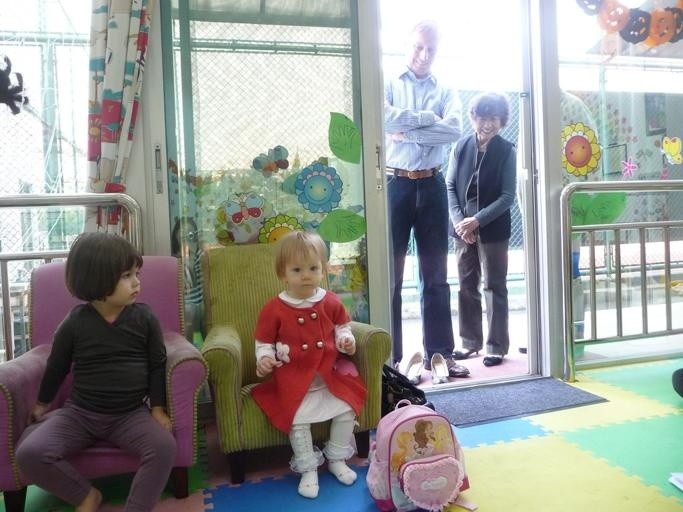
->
[{"xmin": 421, "ymin": 376, "xmax": 609, "ymax": 426}]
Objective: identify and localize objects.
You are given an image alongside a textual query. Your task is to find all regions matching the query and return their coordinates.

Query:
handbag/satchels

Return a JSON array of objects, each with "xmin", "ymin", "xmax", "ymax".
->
[{"xmin": 383, "ymin": 364, "xmax": 435, "ymax": 418}]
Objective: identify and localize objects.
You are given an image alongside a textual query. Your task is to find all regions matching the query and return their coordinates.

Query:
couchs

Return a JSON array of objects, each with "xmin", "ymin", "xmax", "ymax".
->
[
  {"xmin": 200, "ymin": 238, "xmax": 394, "ymax": 485},
  {"xmin": 2, "ymin": 256, "xmax": 210, "ymax": 511}
]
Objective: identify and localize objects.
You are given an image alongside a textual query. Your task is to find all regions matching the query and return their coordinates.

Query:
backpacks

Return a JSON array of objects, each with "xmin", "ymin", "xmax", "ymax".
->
[{"xmin": 366, "ymin": 397, "xmax": 471, "ymax": 511}]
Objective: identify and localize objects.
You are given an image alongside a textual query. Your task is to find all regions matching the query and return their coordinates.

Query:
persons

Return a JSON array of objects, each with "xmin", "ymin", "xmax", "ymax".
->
[
  {"xmin": 519, "ymin": 74, "xmax": 600, "ymax": 363},
  {"xmin": 446, "ymin": 91, "xmax": 517, "ymax": 366},
  {"xmin": 15, "ymin": 232, "xmax": 178, "ymax": 512},
  {"xmin": 382, "ymin": 22, "xmax": 470, "ymax": 374},
  {"xmin": 250, "ymin": 232, "xmax": 368, "ymax": 499}
]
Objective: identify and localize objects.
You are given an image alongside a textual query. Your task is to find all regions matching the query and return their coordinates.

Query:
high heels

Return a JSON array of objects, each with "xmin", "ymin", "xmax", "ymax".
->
[
  {"xmin": 405, "ymin": 351, "xmax": 424, "ymax": 385},
  {"xmin": 483, "ymin": 354, "xmax": 505, "ymax": 367},
  {"xmin": 452, "ymin": 346, "xmax": 480, "ymax": 360}
]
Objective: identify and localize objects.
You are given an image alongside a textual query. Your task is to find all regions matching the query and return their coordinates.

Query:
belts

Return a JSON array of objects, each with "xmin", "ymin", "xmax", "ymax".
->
[{"xmin": 385, "ymin": 166, "xmax": 442, "ymax": 181}]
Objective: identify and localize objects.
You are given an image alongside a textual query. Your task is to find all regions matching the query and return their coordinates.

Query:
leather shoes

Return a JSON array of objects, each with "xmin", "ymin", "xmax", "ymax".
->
[
  {"xmin": 423, "ymin": 352, "xmax": 471, "ymax": 377},
  {"xmin": 430, "ymin": 351, "xmax": 448, "ymax": 384}
]
